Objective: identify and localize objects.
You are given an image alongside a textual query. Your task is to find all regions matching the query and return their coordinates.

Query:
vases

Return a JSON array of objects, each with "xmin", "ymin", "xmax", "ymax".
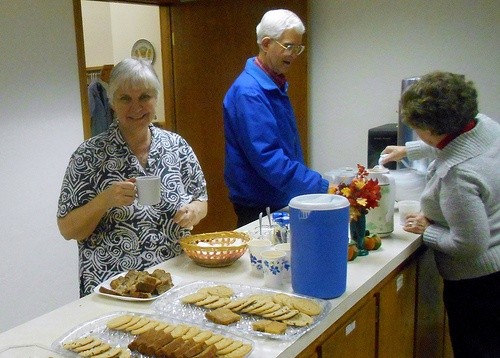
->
[{"xmin": 350, "ymin": 213, "xmax": 369, "ymax": 256}]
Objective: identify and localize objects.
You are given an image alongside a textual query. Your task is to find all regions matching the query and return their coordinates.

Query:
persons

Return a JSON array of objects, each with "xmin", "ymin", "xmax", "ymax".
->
[
  {"xmin": 380, "ymin": 71, "xmax": 500, "ymax": 358},
  {"xmin": 88, "ymin": 65, "xmax": 115, "ymax": 138},
  {"xmin": 222, "ymin": 9, "xmax": 338, "ymax": 228},
  {"xmin": 56, "ymin": 57, "xmax": 209, "ymax": 299}
]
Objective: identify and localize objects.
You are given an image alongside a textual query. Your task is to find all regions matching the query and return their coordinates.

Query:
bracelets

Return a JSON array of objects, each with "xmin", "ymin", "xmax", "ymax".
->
[{"xmin": 425, "ymin": 222, "xmax": 430, "ymax": 229}]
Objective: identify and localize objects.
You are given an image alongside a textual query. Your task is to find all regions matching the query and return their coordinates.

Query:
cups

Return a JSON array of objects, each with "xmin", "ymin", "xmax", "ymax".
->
[
  {"xmin": 272, "ymin": 244, "xmax": 291, "ymax": 279},
  {"xmin": 248, "ymin": 239, "xmax": 273, "ymax": 274},
  {"xmin": 131, "ymin": 176, "xmax": 161, "ymax": 206},
  {"xmin": 262, "ymin": 250, "xmax": 287, "ymax": 287},
  {"xmin": 397, "ymin": 200, "xmax": 421, "ymax": 226}
]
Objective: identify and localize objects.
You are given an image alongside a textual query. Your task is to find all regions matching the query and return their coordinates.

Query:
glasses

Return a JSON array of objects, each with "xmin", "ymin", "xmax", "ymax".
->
[{"xmin": 273, "ymin": 37, "xmax": 305, "ymax": 56}]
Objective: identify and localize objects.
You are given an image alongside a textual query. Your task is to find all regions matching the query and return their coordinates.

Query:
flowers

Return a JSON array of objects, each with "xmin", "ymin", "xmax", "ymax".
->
[{"xmin": 329, "ymin": 165, "xmax": 382, "ymax": 222}]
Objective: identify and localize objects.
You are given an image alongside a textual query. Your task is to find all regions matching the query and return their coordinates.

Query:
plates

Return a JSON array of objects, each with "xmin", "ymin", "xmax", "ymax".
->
[
  {"xmin": 92, "ymin": 272, "xmax": 175, "ymax": 301},
  {"xmin": 130, "ymin": 39, "xmax": 154, "ymax": 65},
  {"xmin": 0, "ymin": 345, "xmax": 67, "ymax": 358},
  {"xmin": 151, "ymin": 280, "xmax": 331, "ymax": 344},
  {"xmin": 53, "ymin": 310, "xmax": 256, "ymax": 358}
]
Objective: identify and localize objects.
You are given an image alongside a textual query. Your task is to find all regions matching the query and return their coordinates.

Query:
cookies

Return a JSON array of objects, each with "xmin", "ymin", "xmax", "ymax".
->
[
  {"xmin": 63, "ymin": 314, "xmax": 253, "ymax": 358},
  {"xmin": 181, "ymin": 285, "xmax": 321, "ymax": 335}
]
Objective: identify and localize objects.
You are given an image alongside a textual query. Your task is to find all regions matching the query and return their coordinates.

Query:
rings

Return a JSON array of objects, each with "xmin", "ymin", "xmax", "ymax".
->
[{"xmin": 409, "ymin": 222, "xmax": 412, "ymax": 227}]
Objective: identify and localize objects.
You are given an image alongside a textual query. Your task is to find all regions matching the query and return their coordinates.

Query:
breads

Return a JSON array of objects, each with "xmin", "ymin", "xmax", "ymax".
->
[{"xmin": 100, "ymin": 269, "xmax": 174, "ymax": 298}]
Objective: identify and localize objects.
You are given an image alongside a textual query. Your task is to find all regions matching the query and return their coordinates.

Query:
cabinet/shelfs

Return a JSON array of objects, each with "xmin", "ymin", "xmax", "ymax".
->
[{"xmin": 0, "ymin": 200, "xmax": 453, "ymax": 358}]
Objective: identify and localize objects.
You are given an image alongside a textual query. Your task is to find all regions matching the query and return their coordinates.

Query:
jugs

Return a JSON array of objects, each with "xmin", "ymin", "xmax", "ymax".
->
[
  {"xmin": 371, "ymin": 155, "xmax": 426, "ymax": 201},
  {"xmin": 365, "ymin": 183, "xmax": 396, "ymax": 236},
  {"xmin": 289, "ymin": 194, "xmax": 348, "ymax": 300}
]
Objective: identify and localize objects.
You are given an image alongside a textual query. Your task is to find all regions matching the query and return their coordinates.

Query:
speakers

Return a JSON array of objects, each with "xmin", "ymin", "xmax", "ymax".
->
[{"xmin": 367, "ymin": 124, "xmax": 397, "ymax": 171}]
